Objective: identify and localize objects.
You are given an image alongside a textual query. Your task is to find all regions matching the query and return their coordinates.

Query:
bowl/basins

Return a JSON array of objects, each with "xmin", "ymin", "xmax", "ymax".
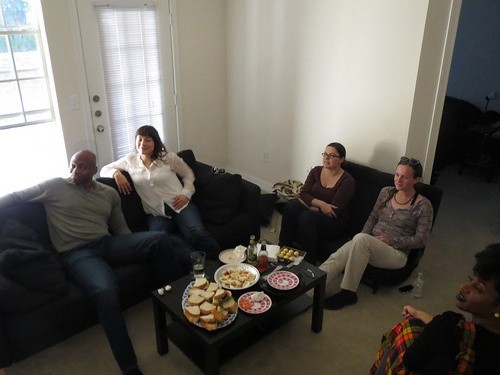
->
[{"xmin": 276, "ymin": 246, "xmax": 306, "ymax": 264}]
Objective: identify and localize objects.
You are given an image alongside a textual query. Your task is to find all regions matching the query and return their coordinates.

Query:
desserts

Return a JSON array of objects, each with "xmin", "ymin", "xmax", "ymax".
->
[{"xmin": 278, "ymin": 248, "xmax": 298, "ymax": 261}]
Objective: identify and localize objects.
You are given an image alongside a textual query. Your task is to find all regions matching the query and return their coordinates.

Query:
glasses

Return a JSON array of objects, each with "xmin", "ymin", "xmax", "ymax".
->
[
  {"xmin": 401, "ymin": 156, "xmax": 421, "ymax": 173},
  {"xmin": 322, "ymin": 153, "xmax": 340, "ymax": 159}
]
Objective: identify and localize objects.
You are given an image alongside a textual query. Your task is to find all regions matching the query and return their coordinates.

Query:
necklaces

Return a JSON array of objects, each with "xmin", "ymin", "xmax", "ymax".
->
[{"xmin": 394, "ymin": 192, "xmax": 415, "ymax": 205}]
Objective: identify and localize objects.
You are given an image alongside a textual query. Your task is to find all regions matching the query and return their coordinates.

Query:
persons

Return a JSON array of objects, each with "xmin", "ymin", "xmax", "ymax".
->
[
  {"xmin": 370, "ymin": 243, "xmax": 500, "ymax": 375},
  {"xmin": 307, "ymin": 157, "xmax": 433, "ymax": 309},
  {"xmin": 100, "ymin": 126, "xmax": 221, "ymax": 268},
  {"xmin": 278, "ymin": 143, "xmax": 360, "ymax": 266},
  {"xmin": 0, "ymin": 150, "xmax": 178, "ymax": 375}
]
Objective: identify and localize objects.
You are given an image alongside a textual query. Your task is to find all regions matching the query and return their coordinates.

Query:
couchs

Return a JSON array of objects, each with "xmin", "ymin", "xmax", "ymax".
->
[
  {"xmin": 0, "ymin": 161, "xmax": 261, "ymax": 368},
  {"xmin": 278, "ymin": 160, "xmax": 444, "ymax": 295}
]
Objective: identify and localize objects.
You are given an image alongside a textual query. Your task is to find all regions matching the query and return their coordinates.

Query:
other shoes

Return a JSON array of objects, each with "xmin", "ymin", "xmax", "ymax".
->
[{"xmin": 324, "ymin": 291, "xmax": 358, "ymax": 311}]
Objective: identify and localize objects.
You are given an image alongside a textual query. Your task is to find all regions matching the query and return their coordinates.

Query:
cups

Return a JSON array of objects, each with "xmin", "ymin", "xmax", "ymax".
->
[{"xmin": 190, "ymin": 250, "xmax": 206, "ymax": 279}]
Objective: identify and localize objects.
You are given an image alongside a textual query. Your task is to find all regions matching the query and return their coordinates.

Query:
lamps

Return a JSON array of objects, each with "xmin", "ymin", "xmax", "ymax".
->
[{"xmin": 483, "ymin": 90, "xmax": 496, "ymax": 113}]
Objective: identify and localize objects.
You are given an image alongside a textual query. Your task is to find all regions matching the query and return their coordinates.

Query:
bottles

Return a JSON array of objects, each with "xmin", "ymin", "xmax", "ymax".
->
[
  {"xmin": 412, "ymin": 271, "xmax": 424, "ymax": 298},
  {"xmin": 246, "ymin": 236, "xmax": 258, "ymax": 266},
  {"xmin": 258, "ymin": 241, "xmax": 268, "ymax": 274}
]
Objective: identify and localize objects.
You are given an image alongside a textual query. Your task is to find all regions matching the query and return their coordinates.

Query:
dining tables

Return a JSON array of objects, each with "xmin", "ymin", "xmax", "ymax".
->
[{"xmin": 150, "ymin": 239, "xmax": 328, "ymax": 375}]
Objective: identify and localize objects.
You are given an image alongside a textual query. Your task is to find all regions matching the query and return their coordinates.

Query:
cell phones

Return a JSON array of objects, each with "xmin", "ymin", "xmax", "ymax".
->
[{"xmin": 398, "ymin": 285, "xmax": 414, "ymax": 294}]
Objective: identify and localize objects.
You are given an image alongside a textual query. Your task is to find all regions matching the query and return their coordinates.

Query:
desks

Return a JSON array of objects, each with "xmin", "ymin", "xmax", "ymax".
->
[{"xmin": 458, "ymin": 121, "xmax": 500, "ymax": 182}]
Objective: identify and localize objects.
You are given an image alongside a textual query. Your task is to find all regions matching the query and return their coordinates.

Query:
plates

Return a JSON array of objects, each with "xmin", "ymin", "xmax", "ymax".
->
[
  {"xmin": 267, "ymin": 271, "xmax": 299, "ymax": 290},
  {"xmin": 238, "ymin": 292, "xmax": 272, "ymax": 314},
  {"xmin": 214, "ymin": 262, "xmax": 260, "ymax": 290},
  {"xmin": 181, "ymin": 281, "xmax": 238, "ymax": 329},
  {"xmin": 219, "ymin": 248, "xmax": 246, "ymax": 264}
]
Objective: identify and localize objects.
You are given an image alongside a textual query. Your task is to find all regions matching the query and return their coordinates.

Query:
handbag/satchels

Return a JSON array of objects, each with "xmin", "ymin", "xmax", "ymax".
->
[{"xmin": 272, "ymin": 180, "xmax": 304, "ymax": 200}]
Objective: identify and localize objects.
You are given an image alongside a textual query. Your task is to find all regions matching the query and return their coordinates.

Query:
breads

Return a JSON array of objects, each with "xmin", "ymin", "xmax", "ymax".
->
[
  {"xmin": 184, "ymin": 277, "xmax": 236, "ymax": 330},
  {"xmin": 220, "ymin": 268, "xmax": 256, "ymax": 288}
]
